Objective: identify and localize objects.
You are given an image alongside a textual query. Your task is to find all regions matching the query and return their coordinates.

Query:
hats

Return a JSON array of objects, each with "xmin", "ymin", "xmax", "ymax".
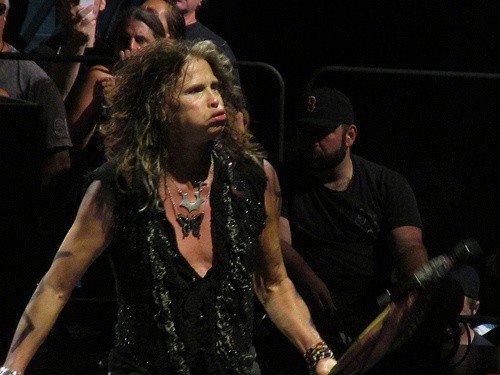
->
[
  {"xmin": 452, "ymin": 263, "xmax": 480, "ymax": 299},
  {"xmin": 293, "ymin": 88, "xmax": 354, "ymax": 127}
]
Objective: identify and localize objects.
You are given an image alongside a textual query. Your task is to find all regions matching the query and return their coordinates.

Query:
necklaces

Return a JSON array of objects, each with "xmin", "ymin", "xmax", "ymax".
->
[{"xmin": 166, "ymin": 158, "xmax": 214, "ymax": 240}]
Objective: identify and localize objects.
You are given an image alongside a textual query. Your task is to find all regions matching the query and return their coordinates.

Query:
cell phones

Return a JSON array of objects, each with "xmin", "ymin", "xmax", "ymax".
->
[{"xmin": 79, "ymin": 0, "xmax": 94, "ymax": 15}]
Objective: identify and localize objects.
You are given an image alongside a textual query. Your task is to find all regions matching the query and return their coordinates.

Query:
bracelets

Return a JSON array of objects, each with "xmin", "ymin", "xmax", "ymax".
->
[
  {"xmin": 302, "ymin": 341, "xmax": 335, "ymax": 370},
  {"xmin": 1, "ymin": 365, "xmax": 25, "ymax": 375}
]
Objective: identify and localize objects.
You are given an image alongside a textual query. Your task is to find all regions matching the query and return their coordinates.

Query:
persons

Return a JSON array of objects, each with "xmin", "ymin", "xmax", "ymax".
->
[
  {"xmin": 0, "ymin": 37, "xmax": 342, "ymax": 375},
  {"xmin": 0, "ymin": 0, "xmax": 500, "ymax": 345}
]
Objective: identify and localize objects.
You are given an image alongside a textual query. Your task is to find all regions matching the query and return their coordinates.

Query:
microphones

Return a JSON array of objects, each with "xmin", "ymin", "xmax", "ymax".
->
[{"xmin": 377, "ymin": 237, "xmax": 483, "ymax": 307}]
